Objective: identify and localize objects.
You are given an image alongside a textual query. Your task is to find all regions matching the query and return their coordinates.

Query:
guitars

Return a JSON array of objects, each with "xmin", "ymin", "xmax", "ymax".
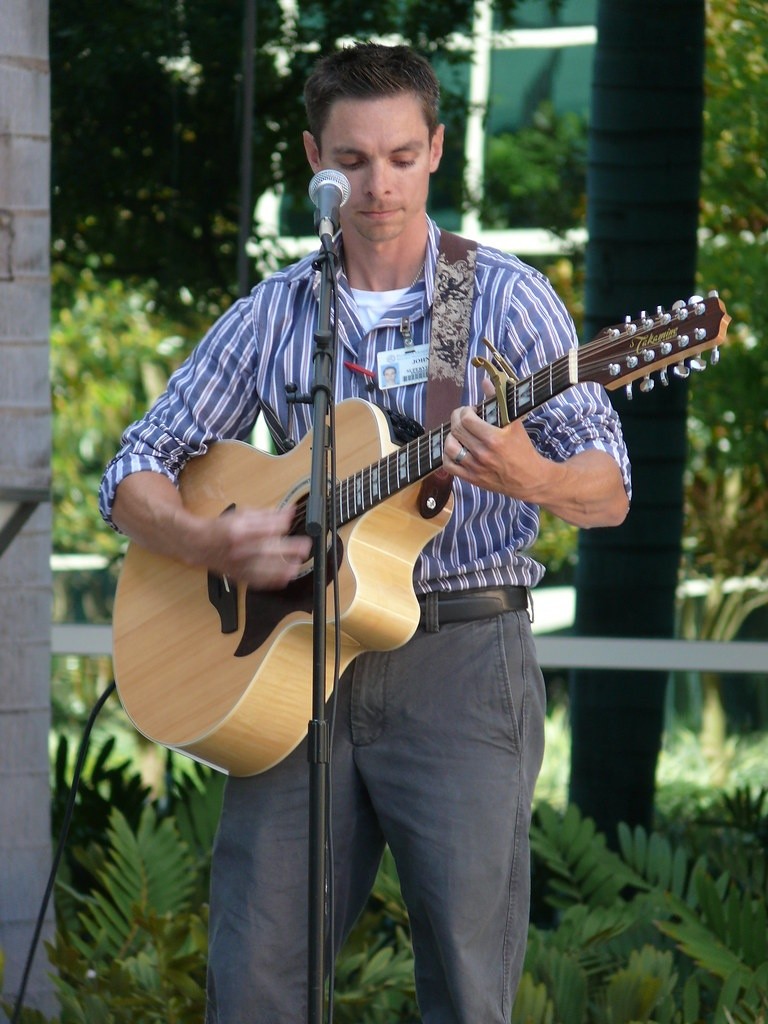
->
[{"xmin": 110, "ymin": 285, "xmax": 736, "ymax": 791}]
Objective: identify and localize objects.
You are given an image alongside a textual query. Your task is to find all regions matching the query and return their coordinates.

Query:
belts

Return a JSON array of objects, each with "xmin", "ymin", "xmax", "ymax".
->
[{"xmin": 418, "ymin": 584, "xmax": 528, "ymax": 626}]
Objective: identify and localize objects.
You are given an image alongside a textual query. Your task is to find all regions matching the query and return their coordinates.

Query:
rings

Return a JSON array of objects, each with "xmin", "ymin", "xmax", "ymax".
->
[{"xmin": 455, "ymin": 447, "xmax": 468, "ymax": 463}]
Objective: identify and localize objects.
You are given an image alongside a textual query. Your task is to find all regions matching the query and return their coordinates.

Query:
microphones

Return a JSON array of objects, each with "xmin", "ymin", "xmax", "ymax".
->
[{"xmin": 308, "ymin": 169, "xmax": 352, "ymax": 253}]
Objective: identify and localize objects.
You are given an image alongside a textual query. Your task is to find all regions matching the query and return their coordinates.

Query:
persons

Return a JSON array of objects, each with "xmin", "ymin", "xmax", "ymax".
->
[
  {"xmin": 96, "ymin": 40, "xmax": 643, "ymax": 1024},
  {"xmin": 382, "ymin": 367, "xmax": 399, "ymax": 387}
]
